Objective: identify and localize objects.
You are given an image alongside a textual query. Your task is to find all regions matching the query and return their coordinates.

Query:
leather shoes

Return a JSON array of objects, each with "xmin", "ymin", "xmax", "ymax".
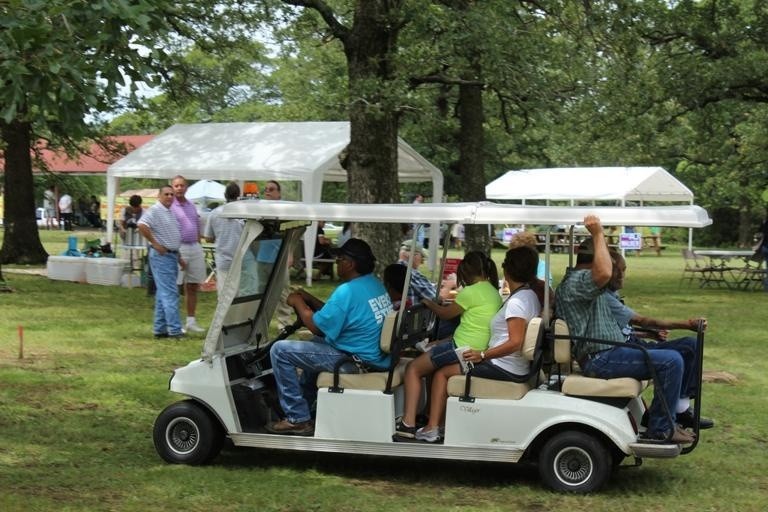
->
[
  {"xmin": 263, "ymin": 419, "xmax": 314, "ymax": 436},
  {"xmin": 263, "ymin": 392, "xmax": 285, "ymax": 418},
  {"xmin": 636, "ymin": 407, "xmax": 712, "ymax": 444}
]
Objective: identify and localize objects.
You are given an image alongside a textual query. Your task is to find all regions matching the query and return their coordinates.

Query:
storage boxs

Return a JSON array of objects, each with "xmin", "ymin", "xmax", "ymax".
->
[
  {"xmin": 46, "ymin": 255, "xmax": 85, "ymax": 282},
  {"xmin": 85, "ymin": 258, "xmax": 134, "ymax": 287}
]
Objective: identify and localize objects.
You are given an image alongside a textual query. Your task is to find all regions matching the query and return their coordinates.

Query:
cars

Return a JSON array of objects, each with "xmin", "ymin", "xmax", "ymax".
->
[
  {"xmin": 322, "ymin": 222, "xmax": 342, "ymax": 233},
  {"xmin": 36, "ymin": 207, "xmax": 65, "ymax": 229}
]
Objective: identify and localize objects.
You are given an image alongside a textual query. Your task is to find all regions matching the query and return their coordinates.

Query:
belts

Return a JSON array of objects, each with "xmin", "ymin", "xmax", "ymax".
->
[{"xmin": 167, "ymin": 249, "xmax": 178, "ymax": 253}]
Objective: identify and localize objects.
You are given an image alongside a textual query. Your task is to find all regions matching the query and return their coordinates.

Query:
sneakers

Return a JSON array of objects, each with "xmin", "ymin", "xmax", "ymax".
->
[
  {"xmin": 185, "ymin": 324, "xmax": 204, "ymax": 332},
  {"xmin": 153, "ymin": 333, "xmax": 167, "ymax": 339},
  {"xmin": 395, "ymin": 414, "xmax": 444, "ymax": 443},
  {"xmin": 168, "ymin": 333, "xmax": 191, "ymax": 341}
]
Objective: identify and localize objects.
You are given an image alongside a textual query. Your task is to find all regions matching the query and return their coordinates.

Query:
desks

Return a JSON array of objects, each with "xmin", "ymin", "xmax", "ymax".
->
[{"xmin": 116, "ymin": 241, "xmax": 216, "ymax": 290}]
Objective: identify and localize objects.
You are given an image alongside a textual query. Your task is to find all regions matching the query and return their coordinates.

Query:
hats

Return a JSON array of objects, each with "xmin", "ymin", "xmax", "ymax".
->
[
  {"xmin": 576, "ymin": 238, "xmax": 616, "ymax": 264},
  {"xmin": 399, "ymin": 239, "xmax": 429, "ymax": 258},
  {"xmin": 327, "ymin": 238, "xmax": 376, "ymax": 262}
]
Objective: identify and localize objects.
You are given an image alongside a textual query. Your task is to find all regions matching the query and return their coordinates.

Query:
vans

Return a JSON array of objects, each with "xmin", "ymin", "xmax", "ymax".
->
[{"xmin": 552, "ymin": 224, "xmax": 642, "ymax": 255}]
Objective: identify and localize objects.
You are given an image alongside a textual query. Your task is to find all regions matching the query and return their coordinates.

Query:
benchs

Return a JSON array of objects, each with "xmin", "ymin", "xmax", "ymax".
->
[
  {"xmin": 303, "ymin": 257, "xmax": 344, "ymax": 281},
  {"xmin": 533, "ymin": 228, "xmax": 667, "ymax": 258}
]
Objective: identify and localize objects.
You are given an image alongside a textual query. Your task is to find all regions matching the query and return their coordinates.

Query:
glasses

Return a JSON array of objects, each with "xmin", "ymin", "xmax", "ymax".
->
[
  {"xmin": 336, "ymin": 256, "xmax": 344, "ymax": 264},
  {"xmin": 265, "ymin": 188, "xmax": 278, "ymax": 192}
]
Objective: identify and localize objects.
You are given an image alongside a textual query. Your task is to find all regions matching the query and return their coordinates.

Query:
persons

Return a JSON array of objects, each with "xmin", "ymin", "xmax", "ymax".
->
[
  {"xmin": 43, "ymin": 185, "xmax": 106, "ymax": 231},
  {"xmin": 253, "ymin": 181, "xmax": 294, "ymax": 335},
  {"xmin": 336, "ymin": 223, "xmax": 353, "ymax": 257},
  {"xmin": 172, "ymin": 176, "xmax": 207, "ymax": 333},
  {"xmin": 204, "ymin": 182, "xmax": 259, "ymax": 299},
  {"xmin": 118, "ymin": 195, "xmax": 144, "ymax": 267},
  {"xmin": 136, "ymin": 185, "xmax": 186, "ymax": 336},
  {"xmin": 313, "ymin": 221, "xmax": 331, "ymax": 271},
  {"xmin": 264, "ymin": 241, "xmax": 395, "ymax": 437}
]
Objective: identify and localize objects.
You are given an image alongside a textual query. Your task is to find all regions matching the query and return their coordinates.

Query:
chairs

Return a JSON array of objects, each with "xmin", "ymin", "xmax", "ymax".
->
[{"xmin": 679, "ymin": 248, "xmax": 768, "ymax": 293}]
